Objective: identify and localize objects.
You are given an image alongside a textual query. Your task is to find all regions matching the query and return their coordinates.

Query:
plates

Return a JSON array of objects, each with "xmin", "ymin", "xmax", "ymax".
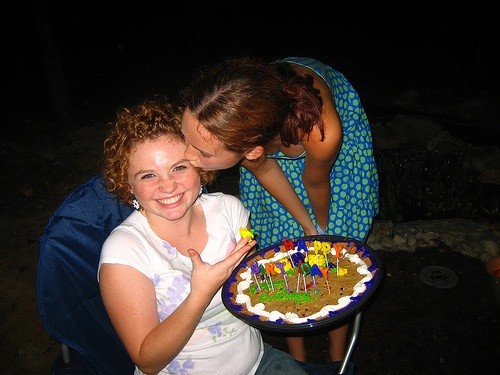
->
[{"xmin": 221, "ymin": 234, "xmax": 382, "ymax": 329}]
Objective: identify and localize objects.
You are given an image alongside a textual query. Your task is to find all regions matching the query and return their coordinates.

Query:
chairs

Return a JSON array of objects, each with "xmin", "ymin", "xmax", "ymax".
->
[{"xmin": 37, "ymin": 170, "xmax": 210, "ymax": 375}]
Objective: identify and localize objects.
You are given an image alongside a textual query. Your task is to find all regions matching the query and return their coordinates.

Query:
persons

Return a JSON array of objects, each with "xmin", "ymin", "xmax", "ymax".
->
[
  {"xmin": 96, "ymin": 98, "xmax": 308, "ymax": 375},
  {"xmin": 180, "ymin": 57, "xmax": 379, "ymax": 242}
]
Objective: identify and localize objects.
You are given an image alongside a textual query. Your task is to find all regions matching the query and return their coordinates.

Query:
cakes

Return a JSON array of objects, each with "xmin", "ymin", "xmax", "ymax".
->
[{"xmin": 224, "ymin": 238, "xmax": 378, "ymax": 325}]
